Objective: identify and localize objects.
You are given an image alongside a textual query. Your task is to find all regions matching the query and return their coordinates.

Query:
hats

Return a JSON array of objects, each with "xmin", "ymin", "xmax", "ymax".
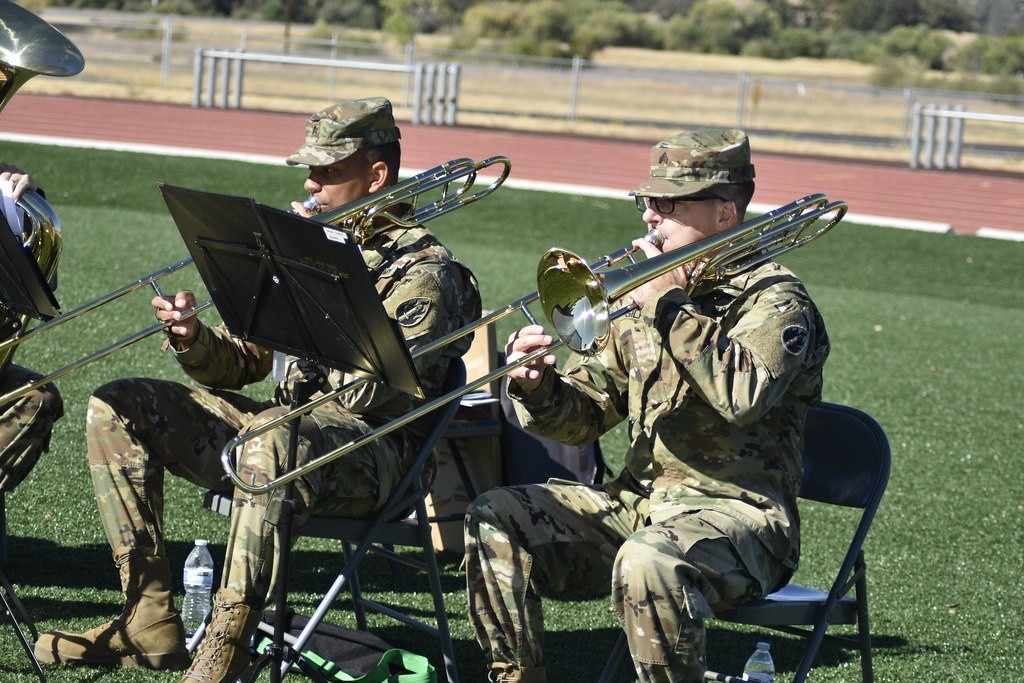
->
[
  {"xmin": 286, "ymin": 97, "xmax": 401, "ymax": 167},
  {"xmin": 629, "ymin": 129, "xmax": 755, "ymax": 198}
]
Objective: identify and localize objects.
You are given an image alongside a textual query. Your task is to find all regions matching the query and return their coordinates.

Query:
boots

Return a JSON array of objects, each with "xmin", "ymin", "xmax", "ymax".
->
[
  {"xmin": 33, "ymin": 554, "xmax": 192, "ymax": 671},
  {"xmin": 179, "ymin": 588, "xmax": 263, "ymax": 683}
]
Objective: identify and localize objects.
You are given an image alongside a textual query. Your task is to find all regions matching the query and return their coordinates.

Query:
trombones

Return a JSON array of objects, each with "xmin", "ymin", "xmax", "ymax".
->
[
  {"xmin": 1, "ymin": 154, "xmax": 515, "ymax": 409},
  {"xmin": 220, "ymin": 188, "xmax": 848, "ymax": 498}
]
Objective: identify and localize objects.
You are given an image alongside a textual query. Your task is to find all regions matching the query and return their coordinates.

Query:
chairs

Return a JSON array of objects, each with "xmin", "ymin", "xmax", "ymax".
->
[
  {"xmin": 202, "ymin": 265, "xmax": 482, "ymax": 682},
  {"xmin": 596, "ymin": 402, "xmax": 893, "ymax": 683}
]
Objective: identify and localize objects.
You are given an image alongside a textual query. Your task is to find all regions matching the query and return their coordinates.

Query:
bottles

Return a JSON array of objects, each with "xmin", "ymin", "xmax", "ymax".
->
[
  {"xmin": 742, "ymin": 642, "xmax": 775, "ymax": 683},
  {"xmin": 180, "ymin": 538, "xmax": 214, "ymax": 638}
]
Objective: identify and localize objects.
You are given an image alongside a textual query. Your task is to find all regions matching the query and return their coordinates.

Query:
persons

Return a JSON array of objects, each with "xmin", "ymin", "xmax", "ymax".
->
[
  {"xmin": 0, "ymin": 164, "xmax": 63, "ymax": 491},
  {"xmin": 34, "ymin": 97, "xmax": 482, "ymax": 683},
  {"xmin": 464, "ymin": 128, "xmax": 830, "ymax": 683}
]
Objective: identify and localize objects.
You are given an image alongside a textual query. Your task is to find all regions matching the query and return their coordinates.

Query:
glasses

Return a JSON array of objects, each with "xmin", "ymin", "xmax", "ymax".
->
[{"xmin": 635, "ymin": 196, "xmax": 736, "ymax": 214}]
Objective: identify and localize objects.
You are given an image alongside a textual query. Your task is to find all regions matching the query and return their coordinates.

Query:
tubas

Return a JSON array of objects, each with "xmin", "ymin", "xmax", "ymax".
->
[{"xmin": 0, "ymin": 0, "xmax": 86, "ymax": 376}]
